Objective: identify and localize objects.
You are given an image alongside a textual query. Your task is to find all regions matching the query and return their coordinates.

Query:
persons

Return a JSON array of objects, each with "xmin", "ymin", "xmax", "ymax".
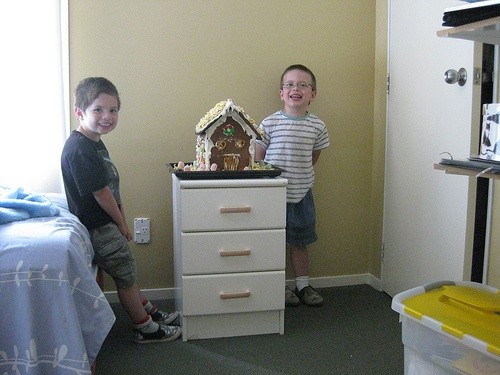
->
[
  {"xmin": 60, "ymin": 77, "xmax": 181, "ymax": 345},
  {"xmin": 252, "ymin": 64, "xmax": 329, "ymax": 306}
]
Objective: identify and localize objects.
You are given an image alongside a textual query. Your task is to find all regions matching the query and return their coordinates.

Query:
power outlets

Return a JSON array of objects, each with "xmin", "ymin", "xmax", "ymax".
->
[{"xmin": 134, "ymin": 218, "xmax": 151, "ymax": 244}]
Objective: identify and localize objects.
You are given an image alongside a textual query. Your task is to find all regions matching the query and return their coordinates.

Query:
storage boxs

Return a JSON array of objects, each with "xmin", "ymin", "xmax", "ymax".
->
[{"xmin": 391, "ymin": 280, "xmax": 500, "ymax": 375}]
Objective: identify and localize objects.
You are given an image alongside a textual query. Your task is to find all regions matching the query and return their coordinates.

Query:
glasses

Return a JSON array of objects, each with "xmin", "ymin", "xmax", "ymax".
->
[{"xmin": 280, "ymin": 82, "xmax": 315, "ymax": 91}]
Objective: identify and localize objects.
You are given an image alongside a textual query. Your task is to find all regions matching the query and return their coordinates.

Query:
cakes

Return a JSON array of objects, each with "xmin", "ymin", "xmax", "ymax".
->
[{"xmin": 174, "ymin": 99, "xmax": 275, "ymax": 171}]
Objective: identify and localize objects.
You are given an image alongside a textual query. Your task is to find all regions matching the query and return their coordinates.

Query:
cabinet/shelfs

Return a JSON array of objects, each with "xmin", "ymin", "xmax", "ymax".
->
[{"xmin": 434, "ymin": 16, "xmax": 500, "ymax": 180}]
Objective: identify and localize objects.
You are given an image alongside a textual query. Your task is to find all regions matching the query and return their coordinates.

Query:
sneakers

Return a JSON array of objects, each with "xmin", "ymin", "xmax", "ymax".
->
[
  {"xmin": 285, "ymin": 286, "xmax": 300, "ymax": 307},
  {"xmin": 151, "ymin": 308, "xmax": 179, "ymax": 325},
  {"xmin": 131, "ymin": 323, "xmax": 181, "ymax": 342},
  {"xmin": 295, "ymin": 284, "xmax": 324, "ymax": 306}
]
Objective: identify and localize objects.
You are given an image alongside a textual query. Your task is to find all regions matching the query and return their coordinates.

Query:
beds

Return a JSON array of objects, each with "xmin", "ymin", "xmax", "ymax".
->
[{"xmin": 0, "ymin": 192, "xmax": 116, "ymax": 375}]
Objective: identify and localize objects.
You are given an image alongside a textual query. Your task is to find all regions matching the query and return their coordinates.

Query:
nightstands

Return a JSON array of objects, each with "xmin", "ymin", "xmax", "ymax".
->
[{"xmin": 172, "ymin": 173, "xmax": 289, "ymax": 342}]
